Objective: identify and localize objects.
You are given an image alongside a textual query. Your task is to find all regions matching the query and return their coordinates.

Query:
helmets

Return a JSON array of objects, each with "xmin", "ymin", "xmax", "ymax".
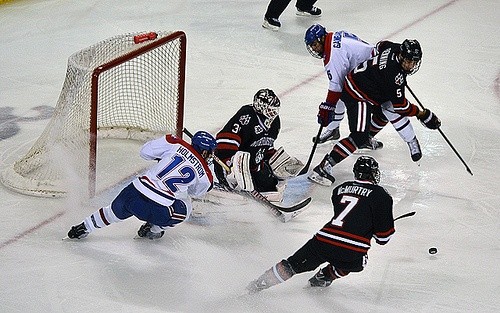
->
[
  {"xmin": 253, "ymin": 88, "xmax": 281, "ymax": 125},
  {"xmin": 353, "ymin": 157, "xmax": 380, "ymax": 185},
  {"xmin": 192, "ymin": 131, "xmax": 216, "ymax": 160},
  {"xmin": 304, "ymin": 24, "xmax": 326, "ymax": 59},
  {"xmin": 397, "ymin": 39, "xmax": 422, "ymax": 75}
]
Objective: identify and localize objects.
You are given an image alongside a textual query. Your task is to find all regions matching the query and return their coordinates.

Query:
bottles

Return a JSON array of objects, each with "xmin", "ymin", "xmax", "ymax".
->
[{"xmin": 134, "ymin": 32, "xmax": 158, "ymax": 44}]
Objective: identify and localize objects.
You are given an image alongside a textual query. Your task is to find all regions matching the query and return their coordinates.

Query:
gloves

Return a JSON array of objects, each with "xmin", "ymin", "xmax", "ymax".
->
[
  {"xmin": 317, "ymin": 103, "xmax": 335, "ymax": 127},
  {"xmin": 418, "ymin": 109, "xmax": 441, "ymax": 130}
]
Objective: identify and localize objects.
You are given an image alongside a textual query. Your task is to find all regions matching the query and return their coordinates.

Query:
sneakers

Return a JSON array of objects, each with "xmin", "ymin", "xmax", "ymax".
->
[
  {"xmin": 353, "ymin": 137, "xmax": 383, "ymax": 154},
  {"xmin": 312, "ymin": 127, "xmax": 340, "ymax": 148},
  {"xmin": 407, "ymin": 137, "xmax": 422, "ymax": 167},
  {"xmin": 262, "ymin": 15, "xmax": 281, "ymax": 31},
  {"xmin": 306, "ymin": 268, "xmax": 332, "ymax": 291},
  {"xmin": 134, "ymin": 223, "xmax": 164, "ymax": 241},
  {"xmin": 63, "ymin": 222, "xmax": 89, "ymax": 241},
  {"xmin": 296, "ymin": 5, "xmax": 321, "ymax": 17},
  {"xmin": 247, "ymin": 280, "xmax": 267, "ymax": 294},
  {"xmin": 307, "ymin": 155, "xmax": 335, "ymax": 187}
]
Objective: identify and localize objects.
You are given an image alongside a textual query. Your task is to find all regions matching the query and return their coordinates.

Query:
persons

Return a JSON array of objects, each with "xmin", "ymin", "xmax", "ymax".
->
[
  {"xmin": 305, "ymin": 24, "xmax": 422, "ymax": 166},
  {"xmin": 261, "ymin": 0, "xmax": 322, "ymax": 33},
  {"xmin": 214, "ymin": 88, "xmax": 304, "ymax": 202},
  {"xmin": 246, "ymin": 155, "xmax": 396, "ymax": 294},
  {"xmin": 307, "ymin": 38, "xmax": 441, "ymax": 187},
  {"xmin": 63, "ymin": 129, "xmax": 216, "ymax": 242}
]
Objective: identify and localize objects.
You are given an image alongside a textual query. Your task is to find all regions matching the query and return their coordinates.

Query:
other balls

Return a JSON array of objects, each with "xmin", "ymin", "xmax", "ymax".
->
[{"xmin": 429, "ymin": 248, "xmax": 438, "ymax": 254}]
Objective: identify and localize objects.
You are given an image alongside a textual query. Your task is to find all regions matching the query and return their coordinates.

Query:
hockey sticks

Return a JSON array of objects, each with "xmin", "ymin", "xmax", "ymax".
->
[
  {"xmin": 393, "ymin": 211, "xmax": 417, "ymax": 222},
  {"xmin": 297, "ymin": 124, "xmax": 322, "ymax": 176},
  {"xmin": 212, "ymin": 182, "xmax": 312, "ymax": 212},
  {"xmin": 182, "ymin": 128, "xmax": 313, "ymax": 224},
  {"xmin": 405, "ymin": 84, "xmax": 474, "ymax": 176}
]
